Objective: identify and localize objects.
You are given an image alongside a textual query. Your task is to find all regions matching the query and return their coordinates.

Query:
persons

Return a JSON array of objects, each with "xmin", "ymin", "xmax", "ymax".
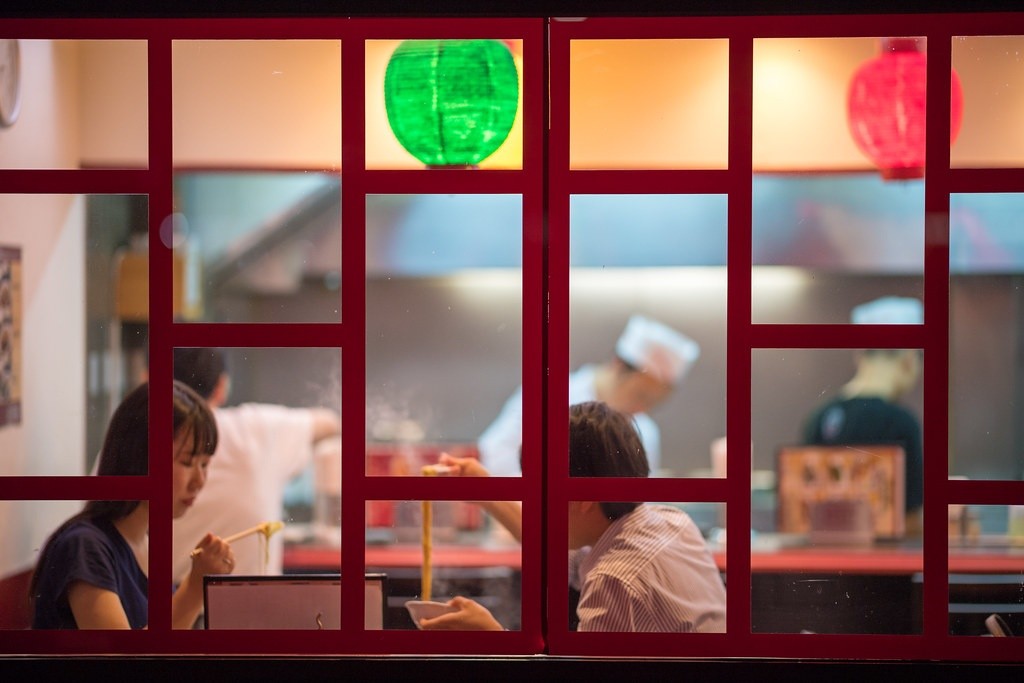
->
[
  {"xmin": 475, "ymin": 314, "xmax": 699, "ymax": 632},
  {"xmin": 28, "ymin": 380, "xmax": 234, "ymax": 630},
  {"xmin": 90, "ymin": 347, "xmax": 341, "ymax": 630},
  {"xmin": 419, "ymin": 401, "xmax": 726, "ymax": 632},
  {"xmin": 803, "ymin": 297, "xmax": 969, "ymax": 540}
]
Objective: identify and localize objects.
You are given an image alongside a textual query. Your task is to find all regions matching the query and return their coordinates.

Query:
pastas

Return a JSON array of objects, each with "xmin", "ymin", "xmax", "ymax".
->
[
  {"xmin": 255, "ymin": 521, "xmax": 272, "ymax": 574},
  {"xmin": 420, "ymin": 466, "xmax": 436, "ymax": 600}
]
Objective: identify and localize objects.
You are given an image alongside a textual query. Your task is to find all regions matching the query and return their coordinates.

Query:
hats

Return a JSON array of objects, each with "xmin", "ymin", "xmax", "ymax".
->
[
  {"xmin": 853, "ymin": 299, "xmax": 923, "ymax": 325},
  {"xmin": 617, "ymin": 319, "xmax": 698, "ymax": 385}
]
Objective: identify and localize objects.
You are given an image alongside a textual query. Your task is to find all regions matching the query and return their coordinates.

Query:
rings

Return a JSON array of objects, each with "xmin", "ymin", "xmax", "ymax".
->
[{"xmin": 223, "ymin": 557, "xmax": 232, "ymax": 565}]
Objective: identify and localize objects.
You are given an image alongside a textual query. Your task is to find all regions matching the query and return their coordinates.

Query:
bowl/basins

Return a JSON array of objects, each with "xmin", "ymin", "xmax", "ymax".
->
[{"xmin": 404, "ymin": 601, "xmax": 459, "ymax": 630}]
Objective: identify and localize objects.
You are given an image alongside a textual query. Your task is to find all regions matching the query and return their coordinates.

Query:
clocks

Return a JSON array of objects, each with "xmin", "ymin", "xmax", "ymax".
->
[{"xmin": 1, "ymin": 38, "xmax": 22, "ymax": 125}]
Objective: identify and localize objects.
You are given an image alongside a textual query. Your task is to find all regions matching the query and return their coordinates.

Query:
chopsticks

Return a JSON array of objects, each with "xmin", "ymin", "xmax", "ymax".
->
[
  {"xmin": 190, "ymin": 526, "xmax": 262, "ymax": 558},
  {"xmin": 431, "ymin": 464, "xmax": 451, "ymax": 474}
]
